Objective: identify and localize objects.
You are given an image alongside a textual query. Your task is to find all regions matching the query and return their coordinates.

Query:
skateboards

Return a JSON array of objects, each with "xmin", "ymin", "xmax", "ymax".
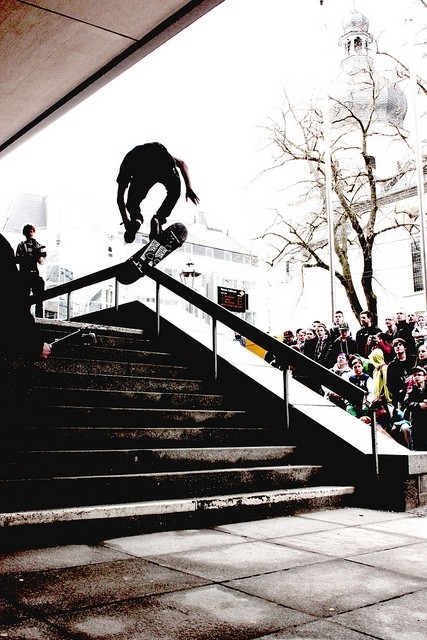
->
[{"xmin": 115, "ymin": 222, "xmax": 188, "ymax": 285}]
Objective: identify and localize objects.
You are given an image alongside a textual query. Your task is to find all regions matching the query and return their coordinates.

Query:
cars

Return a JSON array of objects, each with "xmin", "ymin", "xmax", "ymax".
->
[{"xmin": 242, "ymin": 336, "xmax": 283, "ymax": 370}]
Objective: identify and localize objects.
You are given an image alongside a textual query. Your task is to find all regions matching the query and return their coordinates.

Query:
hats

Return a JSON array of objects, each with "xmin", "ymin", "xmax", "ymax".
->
[
  {"xmin": 317, "ymin": 323, "xmax": 326, "ymax": 329},
  {"xmin": 392, "ymin": 338, "xmax": 405, "ymax": 344},
  {"xmin": 338, "ymin": 323, "xmax": 349, "ymax": 331},
  {"xmin": 351, "ymin": 359, "xmax": 362, "ymax": 364},
  {"xmin": 282, "ymin": 331, "xmax": 292, "ymax": 337},
  {"xmin": 410, "ymin": 366, "xmax": 427, "ymax": 374}
]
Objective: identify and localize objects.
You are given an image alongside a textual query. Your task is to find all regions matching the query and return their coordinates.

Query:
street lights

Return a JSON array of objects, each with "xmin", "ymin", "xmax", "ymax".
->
[
  {"xmin": 178, "ymin": 259, "xmax": 202, "ymax": 313},
  {"xmin": 319, "ymin": 0, "xmax": 374, "ymax": 325}
]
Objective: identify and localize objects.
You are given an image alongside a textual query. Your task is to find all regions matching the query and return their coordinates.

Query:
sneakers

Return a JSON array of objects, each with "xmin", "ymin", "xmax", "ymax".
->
[
  {"xmin": 124, "ymin": 221, "xmax": 141, "ymax": 243},
  {"xmin": 149, "ymin": 216, "xmax": 159, "ymax": 241}
]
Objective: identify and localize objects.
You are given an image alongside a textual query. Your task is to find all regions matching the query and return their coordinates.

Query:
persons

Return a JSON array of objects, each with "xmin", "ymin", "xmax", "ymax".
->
[
  {"xmin": 387, "ymin": 338, "xmax": 418, "ymax": 402},
  {"xmin": 359, "ymin": 401, "xmax": 411, "ymax": 449},
  {"xmin": 345, "ymin": 358, "xmax": 375, "ymax": 417},
  {"xmin": 367, "ymin": 348, "xmax": 393, "ymax": 403},
  {"xmin": 117, "ymin": 143, "xmax": 200, "ymax": 244},
  {"xmin": 288, "ymin": 345, "xmax": 323, "ymax": 396},
  {"xmin": 363, "ymin": 332, "xmax": 393, "ymax": 357},
  {"xmin": 392, "ymin": 310, "xmax": 427, "ymax": 337},
  {"xmin": 348, "ymin": 353, "xmax": 372, "ymax": 379},
  {"xmin": 0, "ymin": 234, "xmax": 53, "ymax": 360},
  {"xmin": 414, "ymin": 345, "xmax": 427, "ymax": 368},
  {"xmin": 384, "ymin": 317, "xmax": 393, "ymax": 334},
  {"xmin": 14, "ymin": 225, "xmax": 47, "ymax": 317},
  {"xmin": 281, "ymin": 319, "xmax": 323, "ymax": 342},
  {"xmin": 403, "ymin": 366, "xmax": 427, "ymax": 451},
  {"xmin": 321, "ymin": 353, "xmax": 357, "ymax": 401},
  {"xmin": 324, "ymin": 311, "xmax": 354, "ymax": 353},
  {"xmin": 358, "ymin": 311, "xmax": 382, "ymax": 355}
]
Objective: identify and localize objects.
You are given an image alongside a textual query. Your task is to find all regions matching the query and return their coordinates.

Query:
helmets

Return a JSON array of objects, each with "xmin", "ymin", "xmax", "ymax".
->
[{"xmin": 22, "ymin": 225, "xmax": 34, "ymax": 231}]
